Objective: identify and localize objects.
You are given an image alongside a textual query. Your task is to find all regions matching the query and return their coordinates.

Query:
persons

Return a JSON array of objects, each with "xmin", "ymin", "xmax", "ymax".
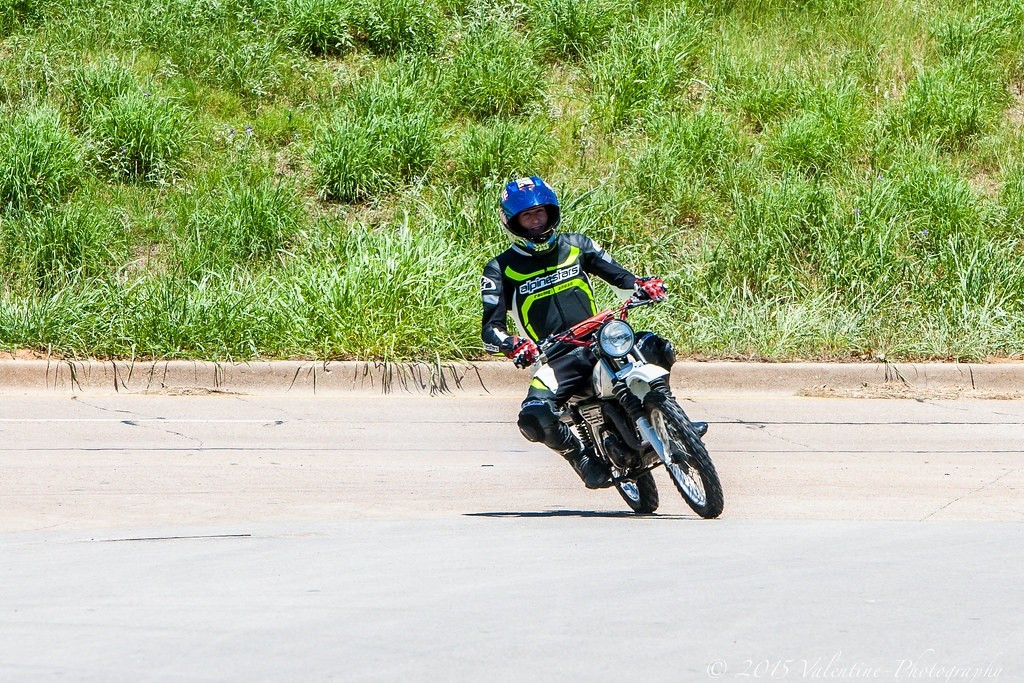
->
[{"xmin": 480, "ymin": 176, "xmax": 668, "ymax": 489}]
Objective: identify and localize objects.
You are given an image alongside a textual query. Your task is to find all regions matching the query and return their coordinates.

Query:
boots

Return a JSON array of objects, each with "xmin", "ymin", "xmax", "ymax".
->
[
  {"xmin": 690, "ymin": 421, "xmax": 708, "ymax": 438},
  {"xmin": 542, "ymin": 419, "xmax": 609, "ymax": 490}
]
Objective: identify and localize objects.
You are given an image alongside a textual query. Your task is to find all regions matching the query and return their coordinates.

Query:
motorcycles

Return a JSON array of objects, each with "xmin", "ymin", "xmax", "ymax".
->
[{"xmin": 509, "ymin": 281, "xmax": 724, "ymax": 520}]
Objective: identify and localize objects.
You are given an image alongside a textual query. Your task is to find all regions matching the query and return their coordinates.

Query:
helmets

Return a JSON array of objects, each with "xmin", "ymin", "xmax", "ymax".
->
[{"xmin": 499, "ymin": 176, "xmax": 561, "ymax": 252}]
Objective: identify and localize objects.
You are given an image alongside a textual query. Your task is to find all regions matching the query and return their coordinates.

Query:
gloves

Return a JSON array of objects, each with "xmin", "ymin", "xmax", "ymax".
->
[
  {"xmin": 634, "ymin": 275, "xmax": 669, "ymax": 301},
  {"xmin": 500, "ymin": 335, "xmax": 540, "ymax": 369}
]
